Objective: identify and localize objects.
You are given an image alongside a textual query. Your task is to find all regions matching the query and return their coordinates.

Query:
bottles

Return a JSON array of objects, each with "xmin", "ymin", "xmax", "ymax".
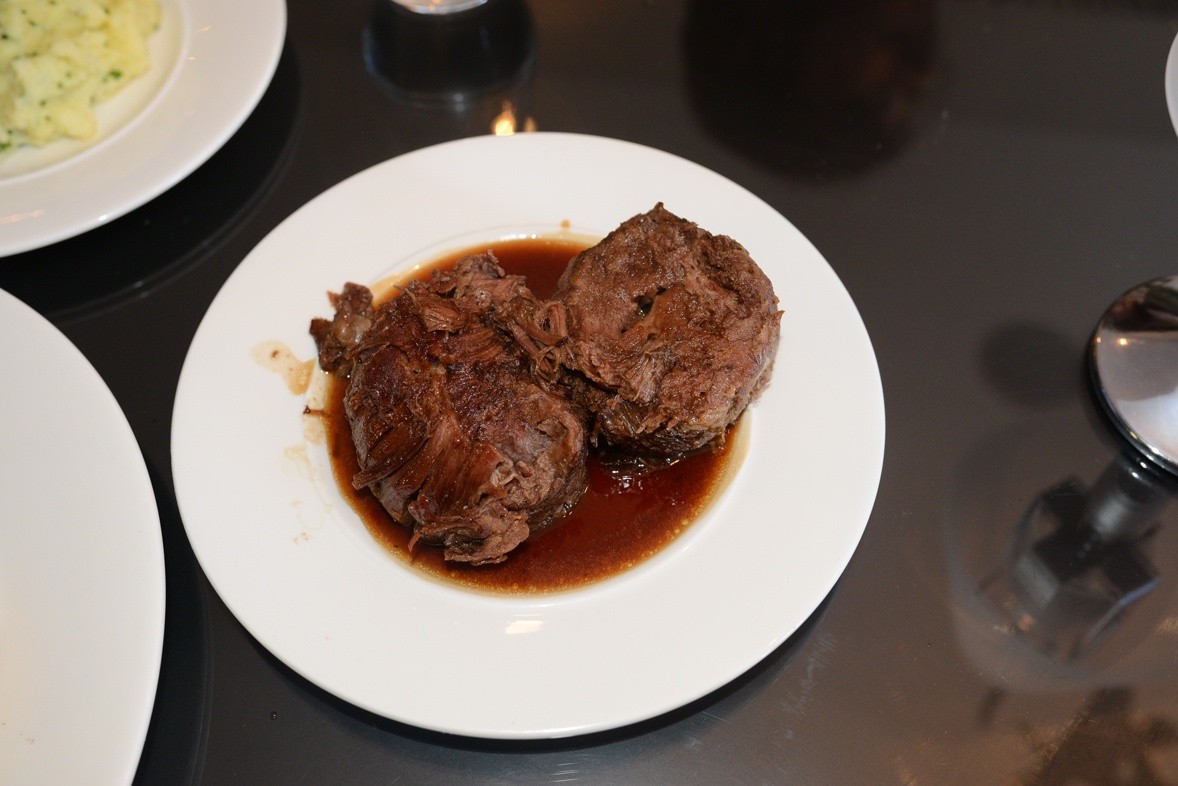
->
[{"xmin": 946, "ymin": 277, "xmax": 1178, "ymax": 698}]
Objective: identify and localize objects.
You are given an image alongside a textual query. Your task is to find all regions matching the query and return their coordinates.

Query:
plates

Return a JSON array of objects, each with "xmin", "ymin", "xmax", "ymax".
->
[
  {"xmin": 171, "ymin": 133, "xmax": 885, "ymax": 741},
  {"xmin": 0, "ymin": 0, "xmax": 288, "ymax": 259},
  {"xmin": 0, "ymin": 284, "xmax": 166, "ymax": 786}
]
